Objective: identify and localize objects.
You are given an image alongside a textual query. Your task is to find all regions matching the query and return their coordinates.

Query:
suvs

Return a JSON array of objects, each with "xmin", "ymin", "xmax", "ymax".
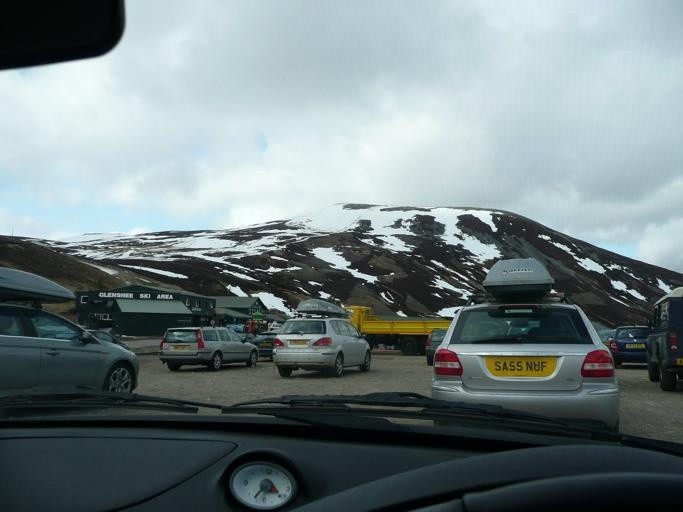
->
[
  {"xmin": 158, "ymin": 299, "xmax": 372, "ymax": 376},
  {"xmin": 0, "ymin": 267, "xmax": 138, "ymax": 399},
  {"xmin": 645, "ymin": 287, "xmax": 682, "ymax": 390},
  {"xmin": 426, "ymin": 255, "xmax": 619, "ymax": 435}
]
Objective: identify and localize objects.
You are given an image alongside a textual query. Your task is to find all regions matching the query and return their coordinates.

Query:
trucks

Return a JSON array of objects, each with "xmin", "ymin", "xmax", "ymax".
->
[{"xmin": 340, "ymin": 305, "xmax": 453, "ymax": 354}]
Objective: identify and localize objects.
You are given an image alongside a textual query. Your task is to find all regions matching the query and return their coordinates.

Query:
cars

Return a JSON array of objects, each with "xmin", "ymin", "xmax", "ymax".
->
[
  {"xmin": 607, "ymin": 325, "xmax": 651, "ymax": 368},
  {"xmin": 426, "ymin": 327, "xmax": 449, "ymax": 365}
]
